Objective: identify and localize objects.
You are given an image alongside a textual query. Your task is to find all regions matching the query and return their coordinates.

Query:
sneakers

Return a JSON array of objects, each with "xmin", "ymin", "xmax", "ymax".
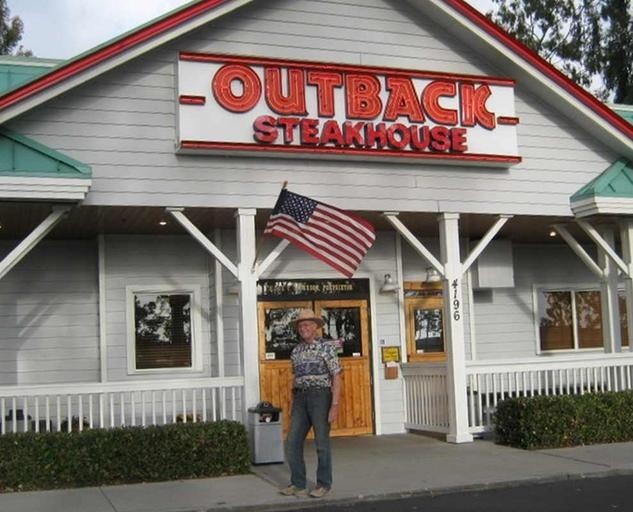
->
[
  {"xmin": 280, "ymin": 483, "xmax": 304, "ymax": 495},
  {"xmin": 309, "ymin": 484, "xmax": 330, "ymax": 497}
]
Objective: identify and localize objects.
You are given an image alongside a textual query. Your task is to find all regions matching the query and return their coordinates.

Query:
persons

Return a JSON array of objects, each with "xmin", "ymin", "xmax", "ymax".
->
[{"xmin": 280, "ymin": 310, "xmax": 341, "ymax": 498}]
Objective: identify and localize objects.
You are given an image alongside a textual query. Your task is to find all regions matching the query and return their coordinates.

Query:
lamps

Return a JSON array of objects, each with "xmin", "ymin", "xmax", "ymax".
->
[
  {"xmin": 380, "ymin": 274, "xmax": 400, "ymax": 293},
  {"xmin": 426, "ymin": 267, "xmax": 442, "ymax": 282}
]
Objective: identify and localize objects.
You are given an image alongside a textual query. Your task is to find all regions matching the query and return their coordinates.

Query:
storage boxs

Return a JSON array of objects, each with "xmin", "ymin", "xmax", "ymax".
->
[{"xmin": 470, "ymin": 239, "xmax": 515, "ymax": 290}]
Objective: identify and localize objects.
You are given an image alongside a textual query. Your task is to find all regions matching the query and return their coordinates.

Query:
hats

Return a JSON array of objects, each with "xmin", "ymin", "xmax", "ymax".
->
[{"xmin": 287, "ymin": 308, "xmax": 324, "ymax": 329}]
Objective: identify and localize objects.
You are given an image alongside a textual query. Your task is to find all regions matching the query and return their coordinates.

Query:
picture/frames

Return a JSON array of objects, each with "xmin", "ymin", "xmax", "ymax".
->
[{"xmin": 381, "ymin": 346, "xmax": 401, "ymax": 363}]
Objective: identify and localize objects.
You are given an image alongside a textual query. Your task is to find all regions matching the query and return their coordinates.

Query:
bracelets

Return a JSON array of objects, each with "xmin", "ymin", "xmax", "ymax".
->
[{"xmin": 331, "ymin": 402, "xmax": 339, "ymax": 405}]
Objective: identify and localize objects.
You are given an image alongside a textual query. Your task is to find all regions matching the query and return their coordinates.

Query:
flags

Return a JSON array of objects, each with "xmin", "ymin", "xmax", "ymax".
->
[{"xmin": 264, "ymin": 189, "xmax": 377, "ymax": 278}]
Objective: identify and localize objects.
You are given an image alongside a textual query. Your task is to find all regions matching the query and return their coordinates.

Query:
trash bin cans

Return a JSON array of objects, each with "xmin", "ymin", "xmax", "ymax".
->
[
  {"xmin": 248, "ymin": 400, "xmax": 284, "ymax": 465},
  {"xmin": 5, "ymin": 409, "xmax": 32, "ymax": 434}
]
